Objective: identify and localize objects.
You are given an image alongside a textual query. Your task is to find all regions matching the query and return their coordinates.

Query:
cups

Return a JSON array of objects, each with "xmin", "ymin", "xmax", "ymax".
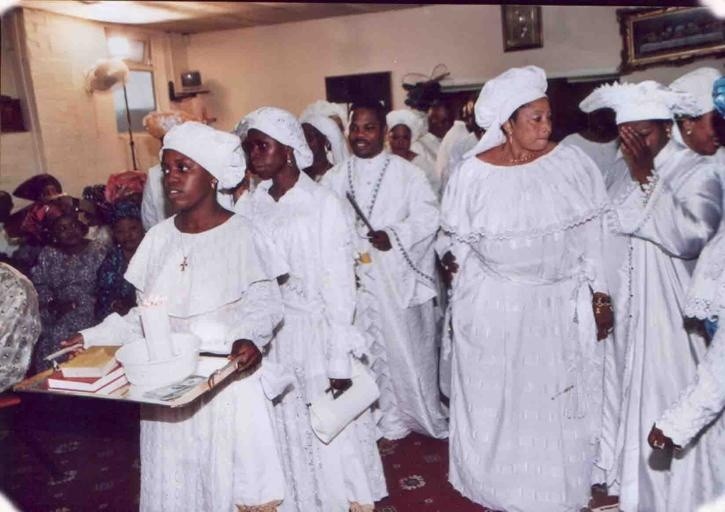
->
[{"xmin": 140, "ymin": 309, "xmax": 171, "ymax": 362}]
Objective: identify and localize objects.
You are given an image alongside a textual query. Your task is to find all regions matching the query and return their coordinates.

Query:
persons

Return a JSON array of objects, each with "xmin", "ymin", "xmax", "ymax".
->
[
  {"xmin": 64, "ymin": 101, "xmax": 448, "ymax": 511},
  {"xmin": 1, "ymin": 176, "xmax": 146, "ymax": 511},
  {"xmin": 437, "ymin": 66, "xmax": 725, "ymax": 511}
]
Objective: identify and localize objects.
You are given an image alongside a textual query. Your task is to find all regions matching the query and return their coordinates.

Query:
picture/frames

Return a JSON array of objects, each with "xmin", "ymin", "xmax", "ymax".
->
[{"xmin": 611, "ymin": 6, "xmax": 724, "ymax": 79}]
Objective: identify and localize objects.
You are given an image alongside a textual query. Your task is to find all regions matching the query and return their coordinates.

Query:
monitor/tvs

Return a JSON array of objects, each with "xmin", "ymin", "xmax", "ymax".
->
[
  {"xmin": 181, "ymin": 71, "xmax": 203, "ymax": 92},
  {"xmin": 325, "ymin": 71, "xmax": 392, "ymax": 114}
]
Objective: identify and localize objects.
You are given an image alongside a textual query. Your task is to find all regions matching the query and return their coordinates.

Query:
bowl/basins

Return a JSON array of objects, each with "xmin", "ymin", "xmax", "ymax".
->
[{"xmin": 116, "ymin": 334, "xmax": 196, "ymax": 389}]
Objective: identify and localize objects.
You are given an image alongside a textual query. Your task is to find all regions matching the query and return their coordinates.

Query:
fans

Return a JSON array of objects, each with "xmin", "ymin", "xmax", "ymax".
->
[{"xmin": 80, "ymin": 58, "xmax": 130, "ymax": 100}]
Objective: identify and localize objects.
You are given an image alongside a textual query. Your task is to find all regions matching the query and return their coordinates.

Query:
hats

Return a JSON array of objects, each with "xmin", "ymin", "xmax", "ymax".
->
[
  {"xmin": 235, "ymin": 106, "xmax": 314, "ymax": 169},
  {"xmin": 13, "ymin": 169, "xmax": 147, "ymax": 242},
  {"xmin": 159, "ymin": 122, "xmax": 246, "ymax": 190},
  {"xmin": 300, "ymin": 98, "xmax": 348, "ymax": 163},
  {"xmin": 579, "ymin": 63, "xmax": 724, "ymax": 126},
  {"xmin": 385, "ymin": 109, "xmax": 422, "ymax": 143},
  {"xmin": 472, "ymin": 64, "xmax": 549, "ymax": 131}
]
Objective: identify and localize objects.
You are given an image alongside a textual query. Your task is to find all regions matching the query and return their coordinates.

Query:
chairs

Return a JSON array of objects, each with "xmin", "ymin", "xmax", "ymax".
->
[{"xmin": 0, "ymin": 332, "xmax": 65, "ymax": 488}]
